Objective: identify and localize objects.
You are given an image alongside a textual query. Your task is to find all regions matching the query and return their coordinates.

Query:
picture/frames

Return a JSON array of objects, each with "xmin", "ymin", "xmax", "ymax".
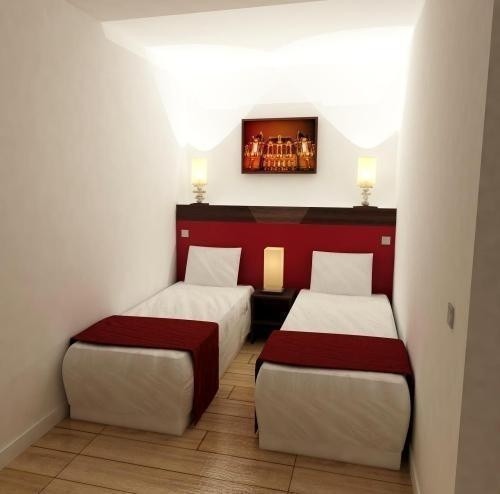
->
[{"xmin": 240, "ymin": 115, "xmax": 319, "ymax": 175}]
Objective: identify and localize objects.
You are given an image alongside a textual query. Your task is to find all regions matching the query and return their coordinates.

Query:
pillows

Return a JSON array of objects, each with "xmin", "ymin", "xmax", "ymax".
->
[
  {"xmin": 181, "ymin": 243, "xmax": 243, "ymax": 288},
  {"xmin": 308, "ymin": 248, "xmax": 375, "ymax": 296}
]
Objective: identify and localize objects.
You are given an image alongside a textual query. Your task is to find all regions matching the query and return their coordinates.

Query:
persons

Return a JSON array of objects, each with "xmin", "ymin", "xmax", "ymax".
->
[
  {"xmin": 295, "ymin": 137, "xmax": 312, "ymax": 168},
  {"xmin": 246, "ymin": 137, "xmax": 265, "ymax": 167}
]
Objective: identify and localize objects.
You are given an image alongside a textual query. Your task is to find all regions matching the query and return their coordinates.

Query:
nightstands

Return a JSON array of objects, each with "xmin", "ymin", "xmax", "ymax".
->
[{"xmin": 248, "ymin": 288, "xmax": 292, "ymax": 341}]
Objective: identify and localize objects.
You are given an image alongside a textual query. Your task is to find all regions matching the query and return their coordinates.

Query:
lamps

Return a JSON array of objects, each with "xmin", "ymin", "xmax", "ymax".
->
[
  {"xmin": 354, "ymin": 153, "xmax": 378, "ymax": 208},
  {"xmin": 190, "ymin": 155, "xmax": 210, "ymax": 205},
  {"xmin": 262, "ymin": 243, "xmax": 286, "ymax": 293}
]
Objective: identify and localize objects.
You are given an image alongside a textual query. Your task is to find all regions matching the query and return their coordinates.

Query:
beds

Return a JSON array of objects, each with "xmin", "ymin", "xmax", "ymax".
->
[
  {"xmin": 248, "ymin": 285, "xmax": 415, "ymax": 472},
  {"xmin": 58, "ymin": 276, "xmax": 252, "ymax": 437}
]
